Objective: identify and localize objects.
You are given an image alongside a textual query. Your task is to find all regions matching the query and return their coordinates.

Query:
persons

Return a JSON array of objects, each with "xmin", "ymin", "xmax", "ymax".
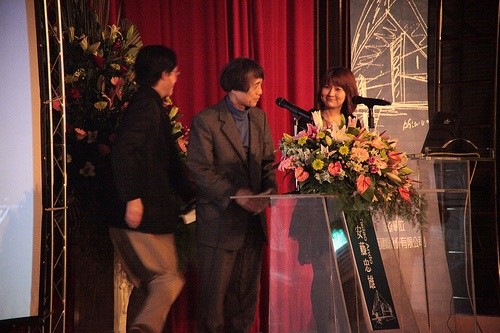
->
[
  {"xmin": 188, "ymin": 57, "xmax": 278, "ymax": 333},
  {"xmin": 106, "ymin": 44, "xmax": 201, "ymax": 333},
  {"xmin": 289, "ymin": 66, "xmax": 368, "ymax": 333}
]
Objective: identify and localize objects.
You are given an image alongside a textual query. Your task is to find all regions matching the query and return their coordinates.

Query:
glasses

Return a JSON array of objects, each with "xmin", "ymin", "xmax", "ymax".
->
[{"xmin": 166, "ymin": 70, "xmax": 180, "ymax": 75}]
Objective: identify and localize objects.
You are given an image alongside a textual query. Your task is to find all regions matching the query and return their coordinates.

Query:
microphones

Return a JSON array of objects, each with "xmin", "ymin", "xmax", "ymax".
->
[
  {"xmin": 276, "ymin": 97, "xmax": 312, "ymax": 120},
  {"xmin": 352, "ymin": 96, "xmax": 391, "ymax": 106}
]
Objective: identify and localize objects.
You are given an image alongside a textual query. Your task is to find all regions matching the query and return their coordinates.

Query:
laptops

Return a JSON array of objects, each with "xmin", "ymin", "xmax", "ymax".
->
[{"xmin": 406, "ymin": 113, "xmax": 459, "ymax": 159}]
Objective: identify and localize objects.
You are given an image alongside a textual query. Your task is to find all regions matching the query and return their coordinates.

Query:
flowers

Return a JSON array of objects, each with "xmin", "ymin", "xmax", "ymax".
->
[
  {"xmin": 277, "ymin": 111, "xmax": 429, "ymax": 223},
  {"xmin": 47, "ymin": 7, "xmax": 188, "ymax": 190}
]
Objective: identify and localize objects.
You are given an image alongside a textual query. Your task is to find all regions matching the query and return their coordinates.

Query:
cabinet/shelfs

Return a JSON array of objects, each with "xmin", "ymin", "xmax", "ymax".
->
[{"xmin": 407, "ymin": 153, "xmax": 495, "ymax": 325}]
